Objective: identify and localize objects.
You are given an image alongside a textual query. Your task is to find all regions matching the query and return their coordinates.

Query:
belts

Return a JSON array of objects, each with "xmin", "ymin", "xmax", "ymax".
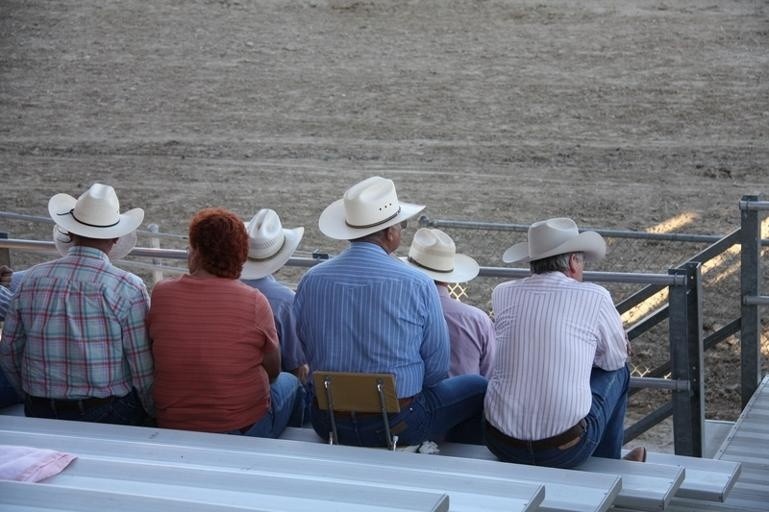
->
[
  {"xmin": 28, "ymin": 394, "xmax": 110, "ymax": 412},
  {"xmin": 334, "ymin": 398, "xmax": 414, "ymax": 416},
  {"xmin": 484, "ymin": 420, "xmax": 584, "ymax": 450}
]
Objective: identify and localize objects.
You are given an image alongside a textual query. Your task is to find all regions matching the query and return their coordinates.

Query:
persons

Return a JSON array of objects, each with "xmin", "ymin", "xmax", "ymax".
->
[{"xmin": 478, "ymin": 217, "xmax": 646, "ymax": 471}]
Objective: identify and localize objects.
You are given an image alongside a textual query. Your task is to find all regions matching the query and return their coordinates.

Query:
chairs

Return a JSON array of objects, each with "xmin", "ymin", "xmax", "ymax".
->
[{"xmin": 311, "ymin": 371, "xmax": 439, "ymax": 455}]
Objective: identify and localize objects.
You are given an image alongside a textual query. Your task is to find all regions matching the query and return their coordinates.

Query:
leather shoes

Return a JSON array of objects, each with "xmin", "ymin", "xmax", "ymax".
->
[{"xmin": 622, "ymin": 447, "xmax": 646, "ymax": 461}]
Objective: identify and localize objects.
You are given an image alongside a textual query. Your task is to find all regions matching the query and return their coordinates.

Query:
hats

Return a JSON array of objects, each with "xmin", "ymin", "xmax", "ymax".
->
[
  {"xmin": 318, "ymin": 176, "xmax": 425, "ymax": 239},
  {"xmin": 238, "ymin": 209, "xmax": 304, "ymax": 281},
  {"xmin": 48, "ymin": 184, "xmax": 144, "ymax": 239},
  {"xmin": 399, "ymin": 228, "xmax": 479, "ymax": 283},
  {"xmin": 52, "ymin": 223, "xmax": 137, "ymax": 259},
  {"xmin": 503, "ymin": 218, "xmax": 605, "ymax": 265}
]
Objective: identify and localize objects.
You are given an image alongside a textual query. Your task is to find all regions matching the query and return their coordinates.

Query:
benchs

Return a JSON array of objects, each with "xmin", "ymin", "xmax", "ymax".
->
[{"xmin": 1, "ymin": 402, "xmax": 745, "ymax": 512}]
